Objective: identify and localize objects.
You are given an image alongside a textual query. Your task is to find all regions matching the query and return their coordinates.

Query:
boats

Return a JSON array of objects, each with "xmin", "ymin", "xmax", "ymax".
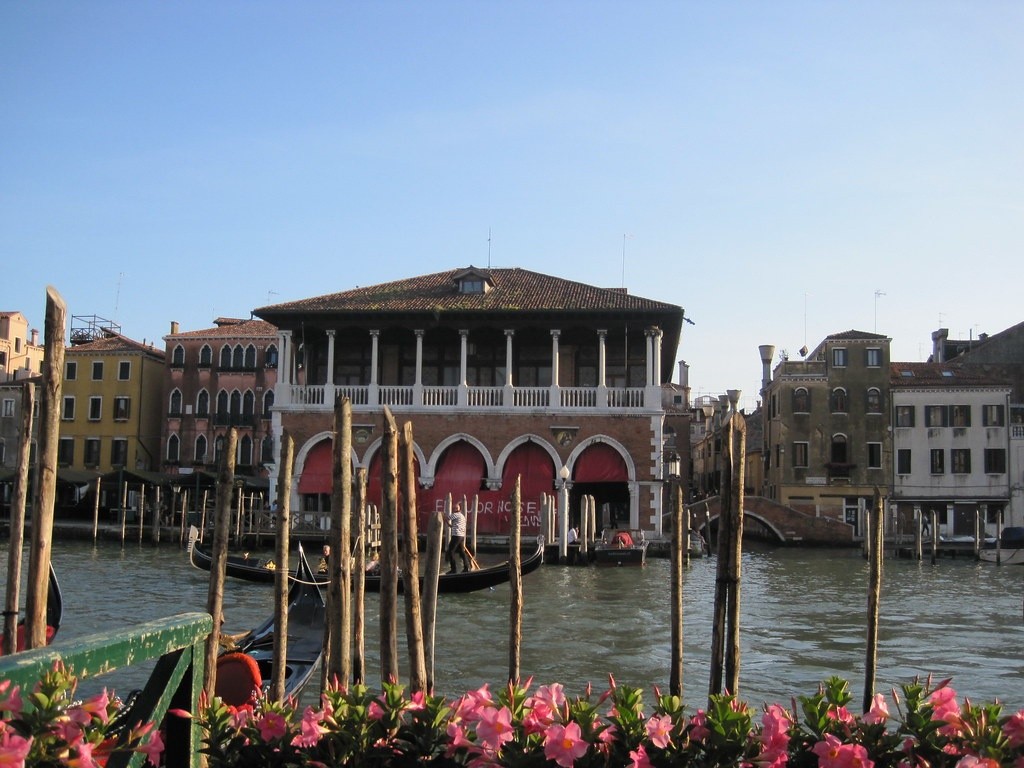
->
[
  {"xmin": 160, "ymin": 540, "xmax": 332, "ymax": 751},
  {"xmin": 187, "ymin": 523, "xmax": 546, "ymax": 592},
  {"xmin": 981, "ymin": 535, "xmax": 1023, "ymax": 566},
  {"xmin": 592, "ymin": 539, "xmax": 648, "ymax": 567},
  {"xmin": 0, "ymin": 558, "xmax": 65, "ymax": 657}
]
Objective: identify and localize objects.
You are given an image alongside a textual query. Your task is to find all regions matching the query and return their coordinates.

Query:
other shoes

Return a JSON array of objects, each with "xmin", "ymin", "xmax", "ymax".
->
[
  {"xmin": 461, "ymin": 568, "xmax": 471, "ymax": 572},
  {"xmin": 446, "ymin": 570, "xmax": 457, "ymax": 574}
]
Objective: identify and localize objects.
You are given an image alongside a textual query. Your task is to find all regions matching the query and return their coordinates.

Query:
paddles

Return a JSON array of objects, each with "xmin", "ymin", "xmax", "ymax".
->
[{"xmin": 441, "ymin": 513, "xmax": 496, "ymax": 591}]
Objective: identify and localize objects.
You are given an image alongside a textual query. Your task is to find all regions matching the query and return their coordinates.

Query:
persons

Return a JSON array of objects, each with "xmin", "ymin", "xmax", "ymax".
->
[
  {"xmin": 323, "ymin": 545, "xmax": 330, "ymax": 566},
  {"xmin": 443, "ymin": 504, "xmax": 471, "ymax": 574},
  {"xmin": 568, "ymin": 524, "xmax": 581, "ymax": 545},
  {"xmin": 921, "ymin": 514, "xmax": 930, "ymax": 538}
]
